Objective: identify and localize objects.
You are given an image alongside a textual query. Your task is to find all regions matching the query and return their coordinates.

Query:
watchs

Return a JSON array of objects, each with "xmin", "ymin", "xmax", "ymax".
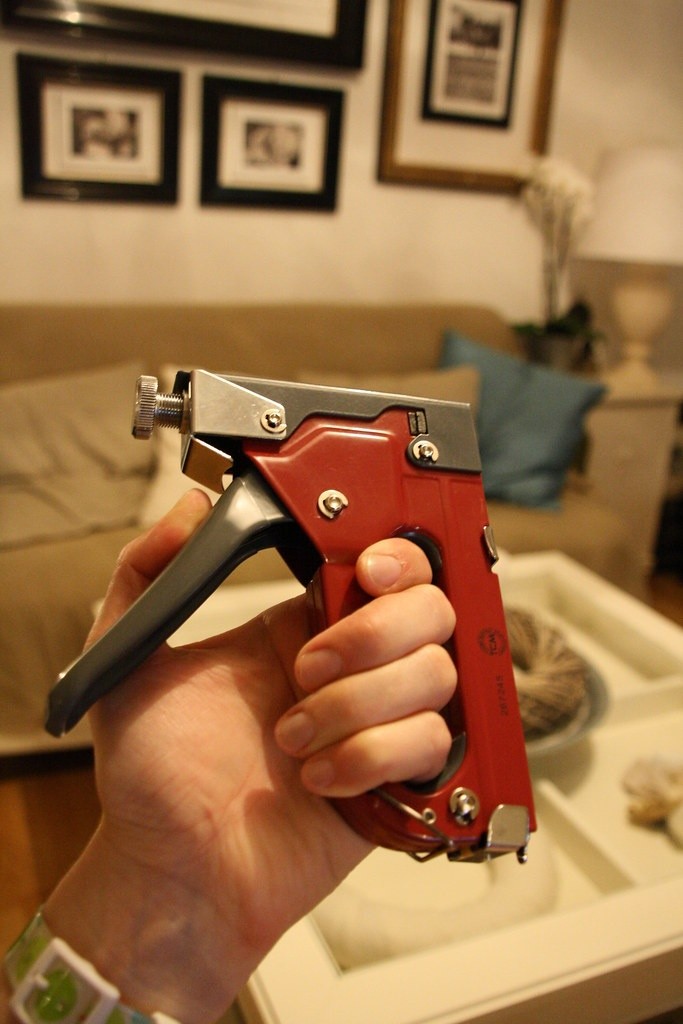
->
[{"xmin": 4, "ymin": 903, "xmax": 179, "ymax": 1024}]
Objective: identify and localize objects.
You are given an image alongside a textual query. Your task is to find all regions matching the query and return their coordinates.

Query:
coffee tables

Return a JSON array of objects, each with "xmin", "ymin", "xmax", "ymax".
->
[{"xmin": 89, "ymin": 550, "xmax": 681, "ymax": 1024}]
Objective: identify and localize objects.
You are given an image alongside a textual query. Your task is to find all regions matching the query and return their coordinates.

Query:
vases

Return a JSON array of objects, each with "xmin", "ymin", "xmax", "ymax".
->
[
  {"xmin": 527, "ymin": 327, "xmax": 597, "ymax": 370},
  {"xmin": 610, "ymin": 281, "xmax": 673, "ymax": 396}
]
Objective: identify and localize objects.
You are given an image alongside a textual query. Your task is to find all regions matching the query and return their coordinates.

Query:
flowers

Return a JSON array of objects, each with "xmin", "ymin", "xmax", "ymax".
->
[{"xmin": 515, "ymin": 148, "xmax": 601, "ymax": 327}]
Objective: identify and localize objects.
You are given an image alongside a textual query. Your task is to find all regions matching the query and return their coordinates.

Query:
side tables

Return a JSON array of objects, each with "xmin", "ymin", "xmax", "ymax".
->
[{"xmin": 583, "ymin": 372, "xmax": 682, "ymax": 593}]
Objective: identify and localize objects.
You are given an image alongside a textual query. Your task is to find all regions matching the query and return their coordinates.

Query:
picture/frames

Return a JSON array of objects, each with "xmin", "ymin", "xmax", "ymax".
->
[
  {"xmin": 15, "ymin": 47, "xmax": 184, "ymax": 209},
  {"xmin": 375, "ymin": 0, "xmax": 570, "ymax": 198},
  {"xmin": 197, "ymin": 70, "xmax": 347, "ymax": 215},
  {"xmin": 0, "ymin": 0, "xmax": 372, "ymax": 73}
]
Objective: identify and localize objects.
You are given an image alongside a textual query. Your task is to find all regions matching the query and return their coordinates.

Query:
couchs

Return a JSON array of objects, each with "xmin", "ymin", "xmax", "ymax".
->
[{"xmin": 1, "ymin": 300, "xmax": 632, "ymax": 760}]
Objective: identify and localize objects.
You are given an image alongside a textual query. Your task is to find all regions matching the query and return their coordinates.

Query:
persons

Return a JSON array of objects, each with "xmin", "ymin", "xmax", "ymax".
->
[{"xmin": 0, "ymin": 488, "xmax": 456, "ymax": 1024}]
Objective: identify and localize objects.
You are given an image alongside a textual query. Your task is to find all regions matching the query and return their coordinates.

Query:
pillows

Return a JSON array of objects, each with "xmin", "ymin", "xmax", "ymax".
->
[
  {"xmin": 1, "ymin": 357, "xmax": 158, "ymax": 549},
  {"xmin": 280, "ymin": 324, "xmax": 607, "ymax": 517}
]
